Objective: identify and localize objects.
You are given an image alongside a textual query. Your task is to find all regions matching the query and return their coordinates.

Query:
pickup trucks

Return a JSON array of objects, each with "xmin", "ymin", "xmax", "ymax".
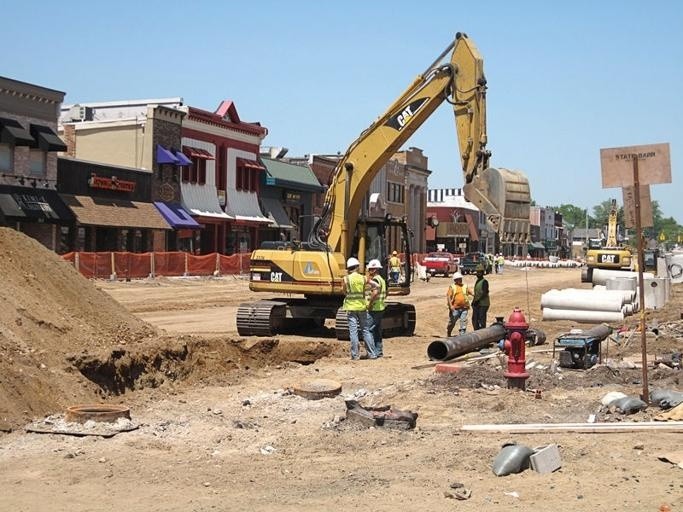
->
[
  {"xmin": 459, "ymin": 252, "xmax": 495, "ymax": 276},
  {"xmin": 420, "ymin": 251, "xmax": 459, "ymax": 277}
]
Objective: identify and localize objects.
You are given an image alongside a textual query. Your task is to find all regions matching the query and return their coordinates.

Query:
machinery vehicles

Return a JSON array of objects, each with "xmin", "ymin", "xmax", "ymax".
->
[
  {"xmin": 234, "ymin": 31, "xmax": 532, "ymax": 337},
  {"xmin": 631, "ymin": 233, "xmax": 661, "ymax": 278},
  {"xmin": 579, "ymin": 198, "xmax": 630, "ymax": 283}
]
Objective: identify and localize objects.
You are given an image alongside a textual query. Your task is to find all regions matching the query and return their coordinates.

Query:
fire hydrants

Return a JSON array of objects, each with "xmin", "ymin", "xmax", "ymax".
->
[{"xmin": 496, "ymin": 306, "xmax": 532, "ymax": 394}]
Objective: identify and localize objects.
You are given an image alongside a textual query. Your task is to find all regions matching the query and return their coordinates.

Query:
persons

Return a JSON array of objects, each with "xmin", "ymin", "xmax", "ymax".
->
[
  {"xmin": 446, "ymin": 271, "xmax": 473, "ymax": 337},
  {"xmin": 340, "ymin": 258, "xmax": 381, "ymax": 361},
  {"xmin": 471, "ymin": 265, "xmax": 491, "ymax": 331},
  {"xmin": 487, "ymin": 253, "xmax": 505, "ymax": 274},
  {"xmin": 364, "ymin": 259, "xmax": 387, "ymax": 358},
  {"xmin": 388, "ymin": 251, "xmax": 402, "ymax": 284}
]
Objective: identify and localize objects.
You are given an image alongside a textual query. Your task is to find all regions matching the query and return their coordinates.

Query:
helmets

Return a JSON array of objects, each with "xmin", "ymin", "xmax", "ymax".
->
[
  {"xmin": 473, "ymin": 264, "xmax": 486, "ymax": 271},
  {"xmin": 366, "ymin": 259, "xmax": 383, "ymax": 270},
  {"xmin": 391, "ymin": 250, "xmax": 397, "ymax": 255},
  {"xmin": 451, "ymin": 271, "xmax": 463, "ymax": 281},
  {"xmin": 345, "ymin": 256, "xmax": 360, "ymax": 269}
]
